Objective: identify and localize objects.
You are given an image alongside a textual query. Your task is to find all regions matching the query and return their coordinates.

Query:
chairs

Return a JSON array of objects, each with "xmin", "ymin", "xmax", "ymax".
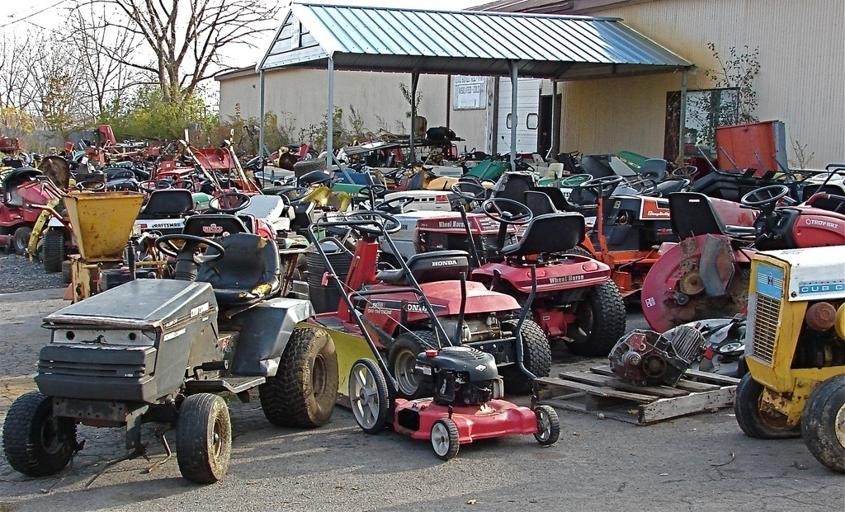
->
[
  {"xmin": 198, "ymin": 232, "xmax": 282, "ymax": 309},
  {"xmin": 375, "ymin": 153, "xmax": 684, "ymax": 287},
  {"xmin": 140, "ymin": 189, "xmax": 194, "ymax": 217}
]
